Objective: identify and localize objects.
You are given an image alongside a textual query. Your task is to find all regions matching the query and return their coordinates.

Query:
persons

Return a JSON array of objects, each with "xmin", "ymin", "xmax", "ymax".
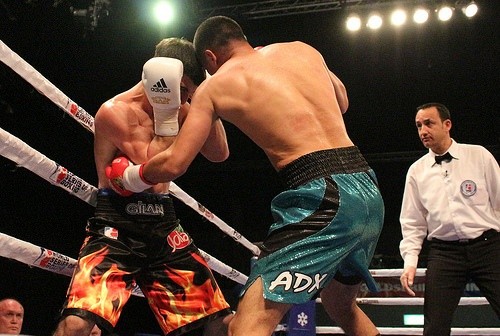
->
[
  {"xmin": 104, "ymin": 17, "xmax": 385, "ymax": 336},
  {"xmin": 398, "ymin": 102, "xmax": 500, "ymax": 336},
  {"xmin": 0, "ymin": 298, "xmax": 24, "ymax": 336},
  {"xmin": 88, "ymin": 323, "xmax": 101, "ymax": 336},
  {"xmin": 53, "ymin": 38, "xmax": 232, "ymax": 336}
]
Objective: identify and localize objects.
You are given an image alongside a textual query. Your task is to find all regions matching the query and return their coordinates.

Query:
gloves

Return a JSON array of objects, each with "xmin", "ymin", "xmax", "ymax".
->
[
  {"xmin": 104, "ymin": 152, "xmax": 158, "ymax": 197},
  {"xmin": 141, "ymin": 57, "xmax": 184, "ymax": 136}
]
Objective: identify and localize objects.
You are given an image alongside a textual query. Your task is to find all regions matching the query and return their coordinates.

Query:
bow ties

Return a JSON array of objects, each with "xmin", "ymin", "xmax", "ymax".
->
[{"xmin": 435, "ymin": 152, "xmax": 452, "ymax": 164}]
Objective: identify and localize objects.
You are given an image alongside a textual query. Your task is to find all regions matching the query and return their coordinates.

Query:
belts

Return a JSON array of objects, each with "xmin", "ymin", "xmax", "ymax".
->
[{"xmin": 432, "ymin": 228, "xmax": 495, "ymax": 245}]
